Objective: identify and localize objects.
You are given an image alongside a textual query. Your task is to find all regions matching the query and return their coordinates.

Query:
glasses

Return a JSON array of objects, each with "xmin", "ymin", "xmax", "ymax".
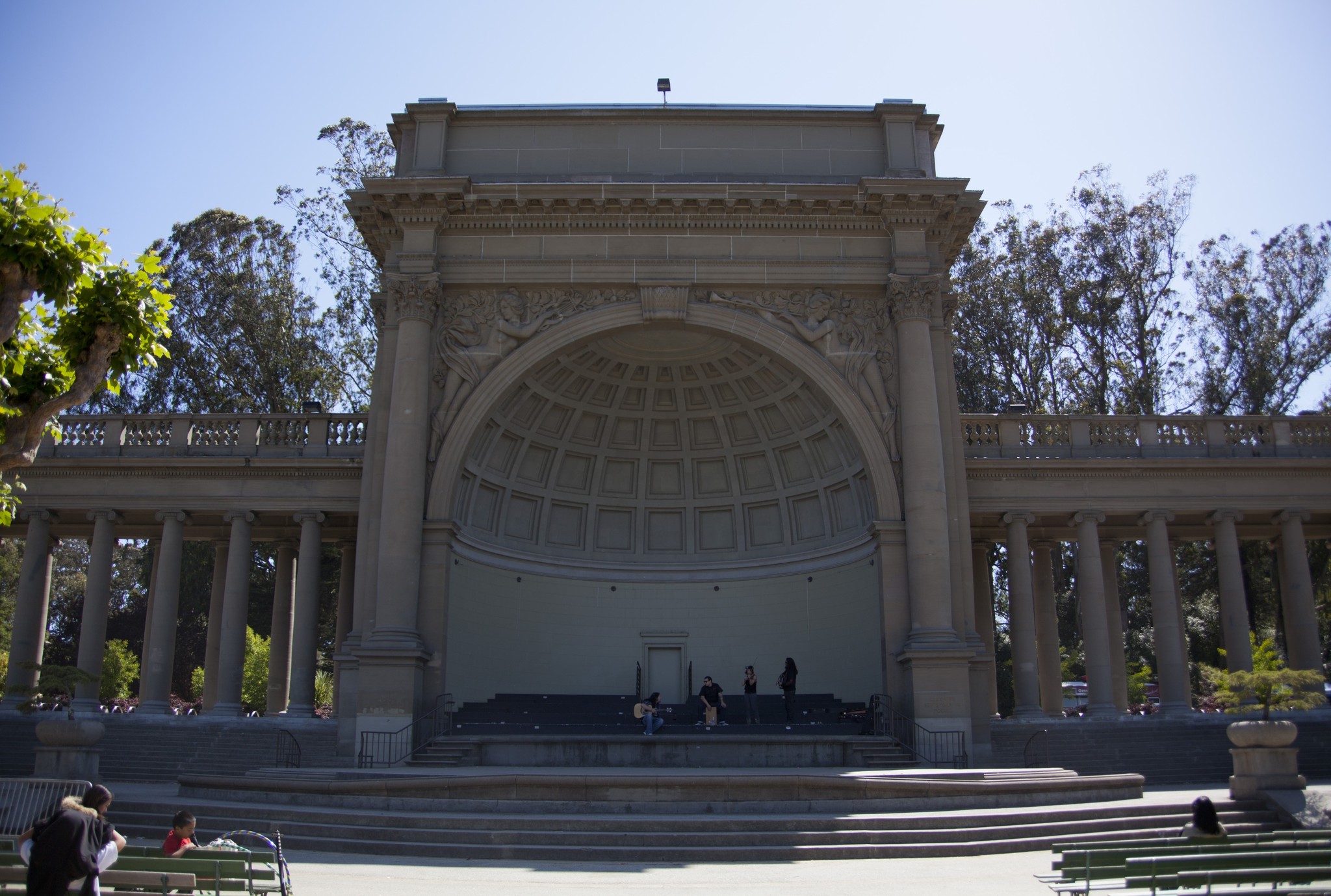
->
[
  {"xmin": 703, "ymin": 681, "xmax": 708, "ymax": 683},
  {"xmin": 785, "ymin": 661, "xmax": 787, "ymax": 664}
]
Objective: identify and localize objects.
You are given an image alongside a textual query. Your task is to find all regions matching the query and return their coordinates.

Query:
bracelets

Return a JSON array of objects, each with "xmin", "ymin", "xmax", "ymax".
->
[{"xmin": 651, "ymin": 708, "xmax": 653, "ymax": 711}]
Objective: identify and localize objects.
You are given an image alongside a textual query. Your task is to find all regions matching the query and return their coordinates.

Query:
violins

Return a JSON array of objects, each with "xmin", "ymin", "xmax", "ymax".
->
[{"xmin": 746, "ymin": 673, "xmax": 754, "ymax": 680}]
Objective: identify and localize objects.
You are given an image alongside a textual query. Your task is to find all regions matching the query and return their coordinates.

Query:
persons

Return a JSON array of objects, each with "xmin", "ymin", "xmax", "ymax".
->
[
  {"xmin": 19, "ymin": 784, "xmax": 205, "ymax": 896},
  {"xmin": 642, "ymin": 692, "xmax": 664, "ymax": 736},
  {"xmin": 1156, "ymin": 796, "xmax": 1228, "ymax": 891},
  {"xmin": 774, "ymin": 657, "xmax": 798, "ymax": 724},
  {"xmin": 742, "ymin": 666, "xmax": 760, "ymax": 725},
  {"xmin": 695, "ymin": 676, "xmax": 729, "ymax": 726}
]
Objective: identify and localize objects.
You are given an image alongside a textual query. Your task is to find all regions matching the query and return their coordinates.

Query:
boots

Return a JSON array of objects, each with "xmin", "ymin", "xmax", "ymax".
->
[
  {"xmin": 755, "ymin": 718, "xmax": 760, "ymax": 725},
  {"xmin": 746, "ymin": 718, "xmax": 751, "ymax": 725}
]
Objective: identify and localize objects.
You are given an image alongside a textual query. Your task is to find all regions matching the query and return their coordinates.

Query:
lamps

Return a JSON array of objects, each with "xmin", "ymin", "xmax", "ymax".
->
[
  {"xmin": 657, "ymin": 78, "xmax": 671, "ymax": 103},
  {"xmin": 303, "ymin": 401, "xmax": 322, "ymax": 413},
  {"xmin": 1008, "ymin": 404, "xmax": 1026, "ymax": 413}
]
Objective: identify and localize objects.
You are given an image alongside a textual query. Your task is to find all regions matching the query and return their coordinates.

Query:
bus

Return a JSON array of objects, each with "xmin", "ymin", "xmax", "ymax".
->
[{"xmin": 1063, "ymin": 681, "xmax": 1159, "ymax": 710}]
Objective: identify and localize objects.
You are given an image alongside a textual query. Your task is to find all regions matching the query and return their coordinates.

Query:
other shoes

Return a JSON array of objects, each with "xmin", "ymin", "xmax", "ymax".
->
[
  {"xmin": 644, "ymin": 732, "xmax": 646, "ymax": 735},
  {"xmin": 648, "ymin": 733, "xmax": 652, "ymax": 735},
  {"xmin": 718, "ymin": 722, "xmax": 728, "ymax": 725},
  {"xmin": 783, "ymin": 721, "xmax": 793, "ymax": 724},
  {"xmin": 695, "ymin": 721, "xmax": 703, "ymax": 725}
]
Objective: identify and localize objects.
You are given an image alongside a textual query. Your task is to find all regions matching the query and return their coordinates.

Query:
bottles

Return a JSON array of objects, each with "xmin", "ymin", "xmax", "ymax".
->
[{"xmin": 712, "ymin": 717, "xmax": 714, "ymax": 725}]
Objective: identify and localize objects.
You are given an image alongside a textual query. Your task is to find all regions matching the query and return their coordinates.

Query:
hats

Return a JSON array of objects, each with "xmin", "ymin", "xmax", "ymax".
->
[{"xmin": 745, "ymin": 666, "xmax": 754, "ymax": 670}]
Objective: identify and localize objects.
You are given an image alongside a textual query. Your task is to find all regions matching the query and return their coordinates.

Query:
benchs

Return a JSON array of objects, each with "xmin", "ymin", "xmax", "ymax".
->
[
  {"xmin": 0, "ymin": 839, "xmax": 282, "ymax": 896},
  {"xmin": 1036, "ymin": 831, "xmax": 1331, "ymax": 896}
]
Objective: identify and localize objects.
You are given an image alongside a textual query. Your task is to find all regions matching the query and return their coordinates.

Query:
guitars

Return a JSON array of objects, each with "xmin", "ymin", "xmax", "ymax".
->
[
  {"xmin": 776, "ymin": 675, "xmax": 785, "ymax": 690},
  {"xmin": 634, "ymin": 703, "xmax": 673, "ymax": 719}
]
